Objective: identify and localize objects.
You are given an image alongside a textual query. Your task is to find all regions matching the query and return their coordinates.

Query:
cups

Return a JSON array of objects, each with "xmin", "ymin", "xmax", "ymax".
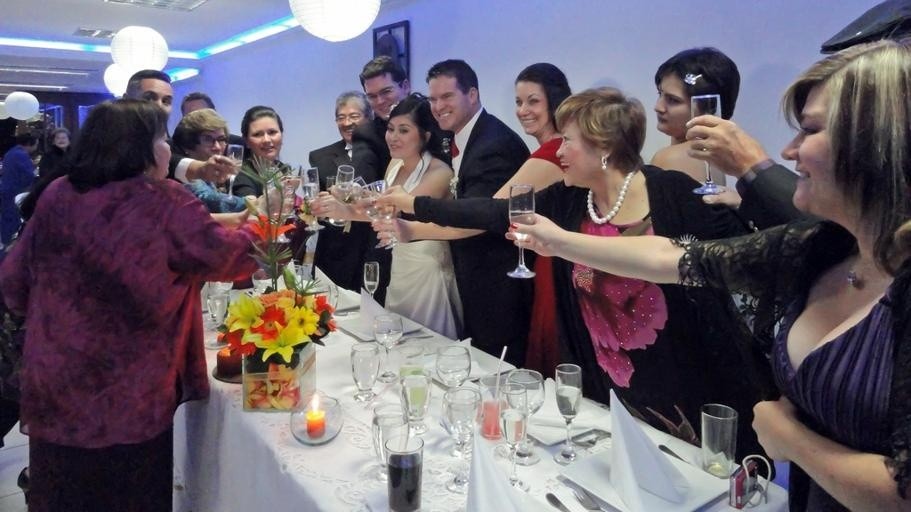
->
[{"xmin": 699, "ymin": 404, "xmax": 739, "ymax": 479}]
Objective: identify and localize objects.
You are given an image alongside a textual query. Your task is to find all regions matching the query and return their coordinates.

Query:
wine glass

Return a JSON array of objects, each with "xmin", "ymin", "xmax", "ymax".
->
[
  {"xmin": 360, "ymin": 259, "xmax": 379, "ymax": 314},
  {"xmin": 687, "ymin": 93, "xmax": 728, "ymax": 194},
  {"xmin": 262, "ymin": 162, "xmax": 399, "ymax": 252},
  {"xmin": 504, "ymin": 184, "xmax": 538, "ymax": 280},
  {"xmin": 504, "ymin": 368, "xmax": 544, "ymax": 466},
  {"xmin": 349, "ymin": 314, "xmax": 493, "ymax": 512},
  {"xmin": 497, "ymin": 382, "xmax": 529, "ymax": 495},
  {"xmin": 552, "ymin": 365, "xmax": 584, "ymax": 466},
  {"xmin": 224, "ymin": 144, "xmax": 244, "ymax": 199}
]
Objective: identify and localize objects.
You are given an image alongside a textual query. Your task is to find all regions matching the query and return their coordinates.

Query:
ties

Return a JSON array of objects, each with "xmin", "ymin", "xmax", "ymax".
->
[{"xmin": 450, "ymin": 139, "xmax": 459, "ymax": 160}]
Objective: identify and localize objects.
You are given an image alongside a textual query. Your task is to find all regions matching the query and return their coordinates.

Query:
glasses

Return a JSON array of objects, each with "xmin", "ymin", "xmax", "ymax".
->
[
  {"xmin": 365, "ymin": 82, "xmax": 402, "ymax": 100},
  {"xmin": 197, "ymin": 135, "xmax": 229, "ymax": 147}
]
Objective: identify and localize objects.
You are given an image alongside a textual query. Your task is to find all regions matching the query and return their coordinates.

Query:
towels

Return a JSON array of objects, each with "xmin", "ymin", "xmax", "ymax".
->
[
  {"xmin": 437, "ymin": 336, "xmax": 483, "ymax": 378},
  {"xmin": 604, "ymin": 383, "xmax": 693, "ymax": 512},
  {"xmin": 359, "ymin": 286, "xmax": 394, "ymax": 336},
  {"xmin": 463, "ymin": 416, "xmax": 542, "ymax": 511}
]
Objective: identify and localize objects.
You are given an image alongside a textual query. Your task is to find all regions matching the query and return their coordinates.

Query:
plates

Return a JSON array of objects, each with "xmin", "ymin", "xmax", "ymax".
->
[{"xmin": 559, "ymin": 442, "xmax": 730, "ymax": 512}]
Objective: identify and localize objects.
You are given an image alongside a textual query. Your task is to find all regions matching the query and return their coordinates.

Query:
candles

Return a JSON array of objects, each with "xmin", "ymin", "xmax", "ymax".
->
[{"xmin": 305, "ymin": 395, "xmax": 328, "ymax": 438}]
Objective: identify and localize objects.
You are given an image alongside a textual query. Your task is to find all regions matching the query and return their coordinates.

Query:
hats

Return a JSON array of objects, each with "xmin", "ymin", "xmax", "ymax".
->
[{"xmin": 819, "ymin": 1, "xmax": 911, "ymax": 55}]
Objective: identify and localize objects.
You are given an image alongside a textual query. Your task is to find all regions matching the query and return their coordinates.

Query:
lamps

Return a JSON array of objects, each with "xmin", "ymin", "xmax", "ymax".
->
[
  {"xmin": 101, "ymin": 63, "xmax": 134, "ymax": 100},
  {"xmin": 1, "ymin": 92, "xmax": 10, "ymax": 120},
  {"xmin": 109, "ymin": 24, "xmax": 169, "ymax": 75},
  {"xmin": 5, "ymin": 60, "xmax": 39, "ymax": 124},
  {"xmin": 287, "ymin": 0, "xmax": 382, "ymax": 45}
]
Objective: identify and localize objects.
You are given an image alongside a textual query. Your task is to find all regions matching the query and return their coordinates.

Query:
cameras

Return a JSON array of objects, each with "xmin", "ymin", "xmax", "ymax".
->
[{"xmin": 730, "ymin": 460, "xmax": 758, "ymax": 509}]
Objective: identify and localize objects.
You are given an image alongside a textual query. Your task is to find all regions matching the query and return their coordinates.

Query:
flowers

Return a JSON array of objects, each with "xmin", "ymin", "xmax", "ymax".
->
[{"xmin": 213, "ymin": 153, "xmax": 343, "ymax": 407}]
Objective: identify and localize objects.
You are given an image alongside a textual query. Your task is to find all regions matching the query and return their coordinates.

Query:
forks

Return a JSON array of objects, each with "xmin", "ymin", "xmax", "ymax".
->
[{"xmin": 573, "ymin": 488, "xmax": 607, "ymax": 512}]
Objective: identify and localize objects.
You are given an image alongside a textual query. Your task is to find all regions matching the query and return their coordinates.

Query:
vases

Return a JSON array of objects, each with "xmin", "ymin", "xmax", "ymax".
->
[{"xmin": 237, "ymin": 342, "xmax": 317, "ymax": 416}]
